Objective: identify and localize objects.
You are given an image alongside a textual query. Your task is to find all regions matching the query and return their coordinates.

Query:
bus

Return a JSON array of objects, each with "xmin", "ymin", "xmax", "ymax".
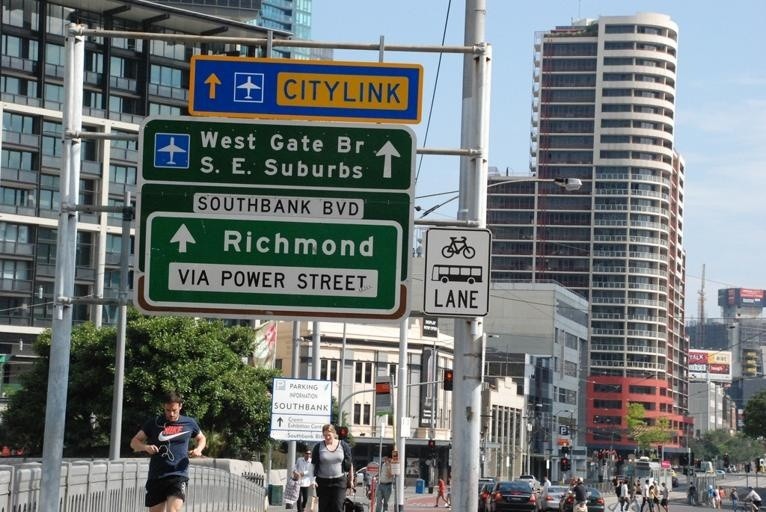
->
[
  {"xmin": 760, "ymin": 457, "xmax": 766, "ymax": 473},
  {"xmin": 614, "ymin": 457, "xmax": 671, "ymax": 496}
]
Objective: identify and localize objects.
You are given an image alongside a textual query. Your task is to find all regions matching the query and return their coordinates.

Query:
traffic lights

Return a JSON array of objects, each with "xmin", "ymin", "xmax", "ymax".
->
[
  {"xmin": 743, "ymin": 351, "xmax": 757, "ymax": 376},
  {"xmin": 429, "ymin": 440, "xmax": 434, "ymax": 449},
  {"xmin": 443, "ymin": 369, "xmax": 454, "ymax": 390},
  {"xmin": 562, "ymin": 457, "xmax": 568, "ymax": 470},
  {"xmin": 562, "ymin": 447, "xmax": 569, "ymax": 453},
  {"xmin": 336, "ymin": 426, "xmax": 347, "ymax": 438},
  {"xmin": 723, "ymin": 452, "xmax": 729, "ymax": 469}
]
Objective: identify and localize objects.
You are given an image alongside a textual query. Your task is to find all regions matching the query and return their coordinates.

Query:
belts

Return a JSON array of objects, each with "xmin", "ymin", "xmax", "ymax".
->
[{"xmin": 380, "ymin": 482, "xmax": 392, "ymax": 486}]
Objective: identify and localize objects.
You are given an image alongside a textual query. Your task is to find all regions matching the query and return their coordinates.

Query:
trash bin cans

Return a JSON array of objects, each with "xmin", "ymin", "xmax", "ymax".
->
[
  {"xmin": 416, "ymin": 479, "xmax": 425, "ymax": 494},
  {"xmin": 268, "ymin": 485, "xmax": 282, "ymax": 506}
]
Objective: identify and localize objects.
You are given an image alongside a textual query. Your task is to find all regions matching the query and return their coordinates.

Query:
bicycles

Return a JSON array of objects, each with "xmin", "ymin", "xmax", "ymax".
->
[{"xmin": 736, "ymin": 497, "xmax": 766, "ymax": 512}]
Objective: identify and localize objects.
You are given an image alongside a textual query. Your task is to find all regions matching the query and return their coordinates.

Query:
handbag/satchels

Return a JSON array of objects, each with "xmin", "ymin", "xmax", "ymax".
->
[
  {"xmin": 342, "ymin": 486, "xmax": 370, "ymax": 512},
  {"xmin": 282, "ymin": 471, "xmax": 301, "ymax": 505},
  {"xmin": 306, "ymin": 495, "xmax": 319, "ymax": 512}
]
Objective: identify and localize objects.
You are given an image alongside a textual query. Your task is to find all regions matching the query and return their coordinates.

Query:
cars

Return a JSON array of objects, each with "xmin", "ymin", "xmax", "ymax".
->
[
  {"xmin": 346, "ymin": 463, "xmax": 368, "ymax": 483},
  {"xmin": 682, "ymin": 462, "xmax": 736, "ymax": 478},
  {"xmin": 671, "ymin": 469, "xmax": 678, "ymax": 488},
  {"xmin": 478, "ymin": 474, "xmax": 604, "ymax": 512}
]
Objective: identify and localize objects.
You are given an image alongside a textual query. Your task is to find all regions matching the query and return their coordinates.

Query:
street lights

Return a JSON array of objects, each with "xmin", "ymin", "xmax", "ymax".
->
[
  {"xmin": 395, "ymin": 174, "xmax": 585, "ymax": 508},
  {"xmin": 564, "ymin": 407, "xmax": 574, "ymax": 478}
]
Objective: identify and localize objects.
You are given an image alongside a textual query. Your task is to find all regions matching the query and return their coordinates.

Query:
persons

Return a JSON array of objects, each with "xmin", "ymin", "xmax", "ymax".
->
[
  {"xmin": 310, "ymin": 423, "xmax": 354, "ymax": 512},
  {"xmin": 129, "ymin": 392, "xmax": 206, "ymax": 512},
  {"xmin": 446, "ymin": 475, "xmax": 452, "ymax": 507},
  {"xmin": 434, "ymin": 477, "xmax": 450, "ymax": 508},
  {"xmin": 571, "ymin": 477, "xmax": 589, "ymax": 512},
  {"xmin": 292, "ymin": 448, "xmax": 314, "ymax": 512},
  {"xmin": 375, "ymin": 454, "xmax": 393, "ymax": 512},
  {"xmin": 612, "ymin": 471, "xmax": 766, "ymax": 512},
  {"xmin": 543, "ymin": 476, "xmax": 552, "ymax": 486}
]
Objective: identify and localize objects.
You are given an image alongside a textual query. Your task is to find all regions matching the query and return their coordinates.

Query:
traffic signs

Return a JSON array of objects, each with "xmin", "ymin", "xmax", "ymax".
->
[
  {"xmin": 141, "ymin": 211, "xmax": 403, "ymax": 312},
  {"xmin": 188, "ymin": 54, "xmax": 424, "ymax": 124},
  {"xmin": 143, "ymin": 118, "xmax": 410, "ymax": 189},
  {"xmin": 272, "ymin": 414, "xmax": 330, "ymax": 431}
]
